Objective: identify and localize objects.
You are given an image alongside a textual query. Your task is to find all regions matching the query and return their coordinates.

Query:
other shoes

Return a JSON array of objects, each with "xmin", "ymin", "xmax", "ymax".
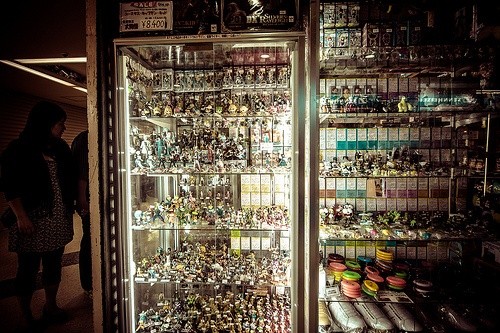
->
[
  {"xmin": 83, "ymin": 289, "xmax": 94, "ymax": 299},
  {"xmin": 25, "ymin": 304, "xmax": 71, "ymax": 330}
]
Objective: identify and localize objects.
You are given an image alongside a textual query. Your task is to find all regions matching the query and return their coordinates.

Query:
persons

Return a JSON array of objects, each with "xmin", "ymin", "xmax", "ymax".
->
[
  {"xmin": 70, "ymin": 130, "xmax": 93, "ymax": 299},
  {"xmin": 0, "ymin": 101, "xmax": 78, "ymax": 333}
]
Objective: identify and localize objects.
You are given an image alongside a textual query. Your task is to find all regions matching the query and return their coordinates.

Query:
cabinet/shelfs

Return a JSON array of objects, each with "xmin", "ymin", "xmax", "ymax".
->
[
  {"xmin": 113, "ymin": 37, "xmax": 297, "ymax": 333},
  {"xmin": 316, "ymin": 0, "xmax": 499, "ymax": 332}
]
